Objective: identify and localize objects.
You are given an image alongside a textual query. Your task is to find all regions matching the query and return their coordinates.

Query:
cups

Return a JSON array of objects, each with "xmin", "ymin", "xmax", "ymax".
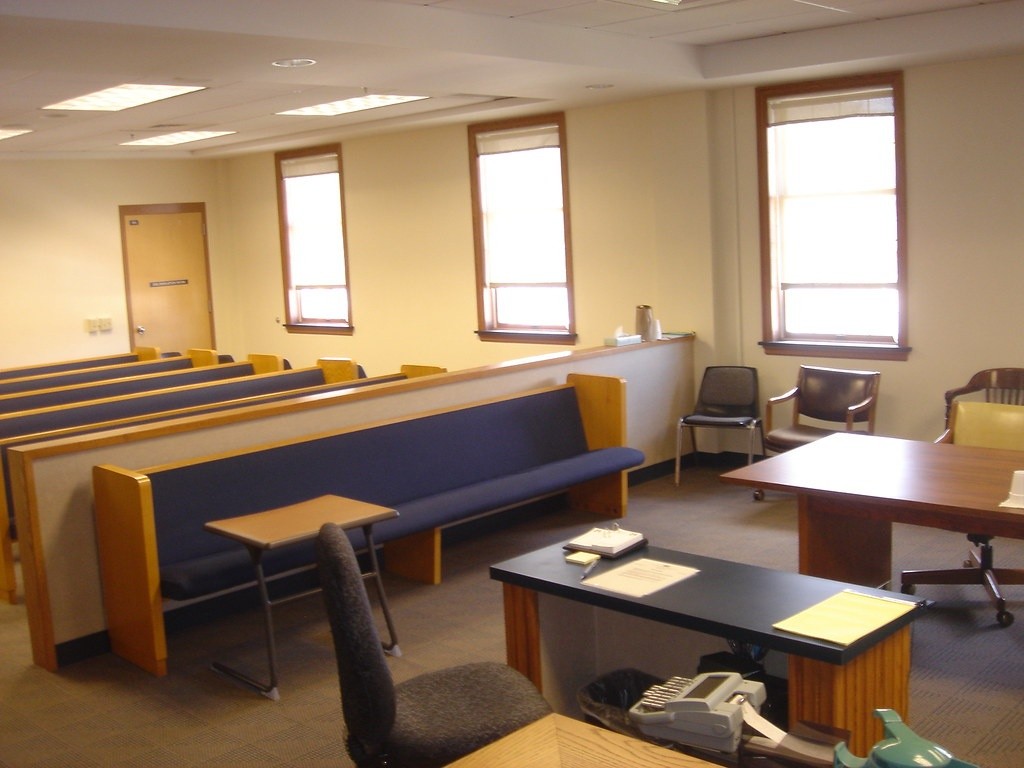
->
[
  {"xmin": 636, "ymin": 304, "xmax": 652, "ymax": 340},
  {"xmin": 1010, "ymin": 471, "xmax": 1024, "ymax": 505},
  {"xmin": 654, "ymin": 320, "xmax": 660, "ymax": 339}
]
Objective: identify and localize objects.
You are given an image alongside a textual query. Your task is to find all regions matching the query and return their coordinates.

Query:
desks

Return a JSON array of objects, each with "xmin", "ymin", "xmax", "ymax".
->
[
  {"xmin": 489, "ymin": 537, "xmax": 936, "ymax": 758},
  {"xmin": 202, "ymin": 493, "xmax": 402, "ymax": 700},
  {"xmin": 718, "ymin": 432, "xmax": 1024, "ymax": 590},
  {"xmin": 442, "ymin": 711, "xmax": 730, "ymax": 768}
]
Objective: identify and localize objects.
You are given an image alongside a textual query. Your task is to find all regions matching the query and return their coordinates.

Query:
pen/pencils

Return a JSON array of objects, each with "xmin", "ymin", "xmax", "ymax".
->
[{"xmin": 580, "ymin": 559, "xmax": 599, "ymax": 581}]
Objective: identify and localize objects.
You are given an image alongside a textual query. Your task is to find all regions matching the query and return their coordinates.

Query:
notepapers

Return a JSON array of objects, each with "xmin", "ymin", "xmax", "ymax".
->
[{"xmin": 564, "ymin": 550, "xmax": 601, "ymax": 565}]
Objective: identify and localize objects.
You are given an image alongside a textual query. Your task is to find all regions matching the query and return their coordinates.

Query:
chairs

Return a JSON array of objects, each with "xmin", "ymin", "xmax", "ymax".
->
[
  {"xmin": 754, "ymin": 365, "xmax": 880, "ymax": 499},
  {"xmin": 323, "ymin": 522, "xmax": 553, "ymax": 768},
  {"xmin": 676, "ymin": 365, "xmax": 767, "ymax": 488},
  {"xmin": 901, "ymin": 368, "xmax": 1024, "ymax": 624}
]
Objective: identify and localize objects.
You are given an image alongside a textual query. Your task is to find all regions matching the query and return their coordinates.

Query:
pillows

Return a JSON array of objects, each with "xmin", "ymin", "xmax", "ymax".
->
[{"xmin": 953, "ymin": 400, "xmax": 1024, "ymax": 450}]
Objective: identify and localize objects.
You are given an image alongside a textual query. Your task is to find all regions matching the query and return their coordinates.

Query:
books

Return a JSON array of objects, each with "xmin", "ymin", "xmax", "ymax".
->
[
  {"xmin": 563, "ymin": 523, "xmax": 648, "ymax": 565},
  {"xmin": 773, "ymin": 589, "xmax": 919, "ymax": 647}
]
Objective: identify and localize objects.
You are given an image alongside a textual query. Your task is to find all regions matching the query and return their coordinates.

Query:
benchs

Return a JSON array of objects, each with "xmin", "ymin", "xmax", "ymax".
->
[{"xmin": 0, "ymin": 348, "xmax": 646, "ymax": 676}]
